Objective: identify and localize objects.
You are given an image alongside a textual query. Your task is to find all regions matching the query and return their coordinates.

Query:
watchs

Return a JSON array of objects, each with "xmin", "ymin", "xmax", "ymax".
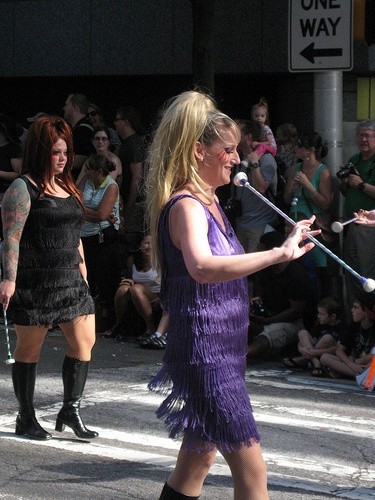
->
[
  {"xmin": 358, "ymin": 182, "xmax": 366, "ymax": 193},
  {"xmin": 249, "ymin": 162, "xmax": 259, "ymax": 169}
]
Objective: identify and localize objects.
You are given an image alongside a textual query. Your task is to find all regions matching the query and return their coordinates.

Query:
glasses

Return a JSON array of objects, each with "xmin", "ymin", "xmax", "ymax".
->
[
  {"xmin": 94, "ymin": 137, "xmax": 110, "ymax": 141},
  {"xmin": 295, "ymin": 142, "xmax": 303, "ymax": 148},
  {"xmin": 85, "ymin": 111, "xmax": 99, "ymax": 117},
  {"xmin": 114, "ymin": 117, "xmax": 130, "ymax": 122}
]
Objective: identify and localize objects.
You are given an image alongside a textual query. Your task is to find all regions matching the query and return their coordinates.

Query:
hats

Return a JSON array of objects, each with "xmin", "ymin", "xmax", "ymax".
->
[{"xmin": 27, "ymin": 112, "xmax": 49, "ymax": 122}]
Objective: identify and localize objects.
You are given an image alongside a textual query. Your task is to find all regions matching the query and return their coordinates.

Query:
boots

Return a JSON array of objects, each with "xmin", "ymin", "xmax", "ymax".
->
[
  {"xmin": 159, "ymin": 481, "xmax": 200, "ymax": 500},
  {"xmin": 12, "ymin": 361, "xmax": 53, "ymax": 440},
  {"xmin": 55, "ymin": 355, "xmax": 99, "ymax": 439}
]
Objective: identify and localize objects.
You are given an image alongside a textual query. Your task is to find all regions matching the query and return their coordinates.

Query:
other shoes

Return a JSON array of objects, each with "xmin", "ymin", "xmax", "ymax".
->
[
  {"xmin": 104, "ymin": 326, "xmax": 126, "ymax": 338},
  {"xmin": 149, "ymin": 336, "xmax": 166, "ymax": 350},
  {"xmin": 137, "ymin": 333, "xmax": 149, "ymax": 340},
  {"xmin": 139, "ymin": 334, "xmax": 157, "ymax": 349}
]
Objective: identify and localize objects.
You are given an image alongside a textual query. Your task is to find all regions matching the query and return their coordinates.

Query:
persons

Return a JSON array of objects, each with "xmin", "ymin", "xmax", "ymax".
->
[
  {"xmin": 0, "ymin": 92, "xmax": 375, "ymax": 392},
  {"xmin": 0, "ymin": 112, "xmax": 99, "ymax": 441},
  {"xmin": 146, "ymin": 90, "xmax": 321, "ymax": 500}
]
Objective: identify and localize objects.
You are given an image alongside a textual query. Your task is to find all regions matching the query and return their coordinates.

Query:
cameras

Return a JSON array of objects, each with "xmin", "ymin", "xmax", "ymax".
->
[{"xmin": 338, "ymin": 163, "xmax": 360, "ymax": 179}]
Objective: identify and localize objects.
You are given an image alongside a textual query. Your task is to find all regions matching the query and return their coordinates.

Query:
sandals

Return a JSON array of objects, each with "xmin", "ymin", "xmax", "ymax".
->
[
  {"xmin": 311, "ymin": 367, "xmax": 324, "ymax": 376},
  {"xmin": 281, "ymin": 357, "xmax": 299, "ymax": 369}
]
